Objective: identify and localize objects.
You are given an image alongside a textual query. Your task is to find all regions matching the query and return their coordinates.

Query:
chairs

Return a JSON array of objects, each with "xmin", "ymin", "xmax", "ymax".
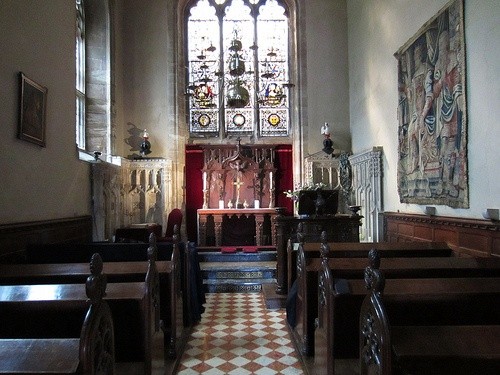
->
[{"xmin": 157, "ymin": 208, "xmax": 184, "ymax": 243}]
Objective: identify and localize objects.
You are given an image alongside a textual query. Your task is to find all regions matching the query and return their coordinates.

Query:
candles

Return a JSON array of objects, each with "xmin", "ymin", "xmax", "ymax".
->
[
  {"xmin": 203, "ymin": 172, "xmax": 207, "ymax": 190},
  {"xmin": 269, "ymin": 171, "xmax": 273, "ymax": 190}
]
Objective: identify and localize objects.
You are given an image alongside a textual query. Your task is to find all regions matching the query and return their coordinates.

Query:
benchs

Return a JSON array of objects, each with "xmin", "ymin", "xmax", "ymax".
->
[
  {"xmin": 283, "ymin": 229, "xmax": 500, "ymax": 375},
  {"xmin": 0, "ymin": 224, "xmax": 207, "ymax": 375}
]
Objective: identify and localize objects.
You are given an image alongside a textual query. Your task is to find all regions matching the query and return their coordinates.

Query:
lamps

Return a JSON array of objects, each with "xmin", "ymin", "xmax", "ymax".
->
[{"xmin": 138, "ymin": 127, "xmax": 154, "ymax": 157}]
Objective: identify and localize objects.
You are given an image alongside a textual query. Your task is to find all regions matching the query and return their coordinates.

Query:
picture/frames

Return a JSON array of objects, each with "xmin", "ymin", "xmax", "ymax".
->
[{"xmin": 16, "ymin": 71, "xmax": 50, "ymax": 149}]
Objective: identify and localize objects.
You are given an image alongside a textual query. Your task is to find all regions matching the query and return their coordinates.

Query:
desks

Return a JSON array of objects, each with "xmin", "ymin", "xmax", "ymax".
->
[
  {"xmin": 196, "ymin": 207, "xmax": 287, "ymax": 247},
  {"xmin": 276, "ymin": 214, "xmax": 365, "ymax": 243},
  {"xmin": 114, "ymin": 223, "xmax": 162, "ymax": 243}
]
